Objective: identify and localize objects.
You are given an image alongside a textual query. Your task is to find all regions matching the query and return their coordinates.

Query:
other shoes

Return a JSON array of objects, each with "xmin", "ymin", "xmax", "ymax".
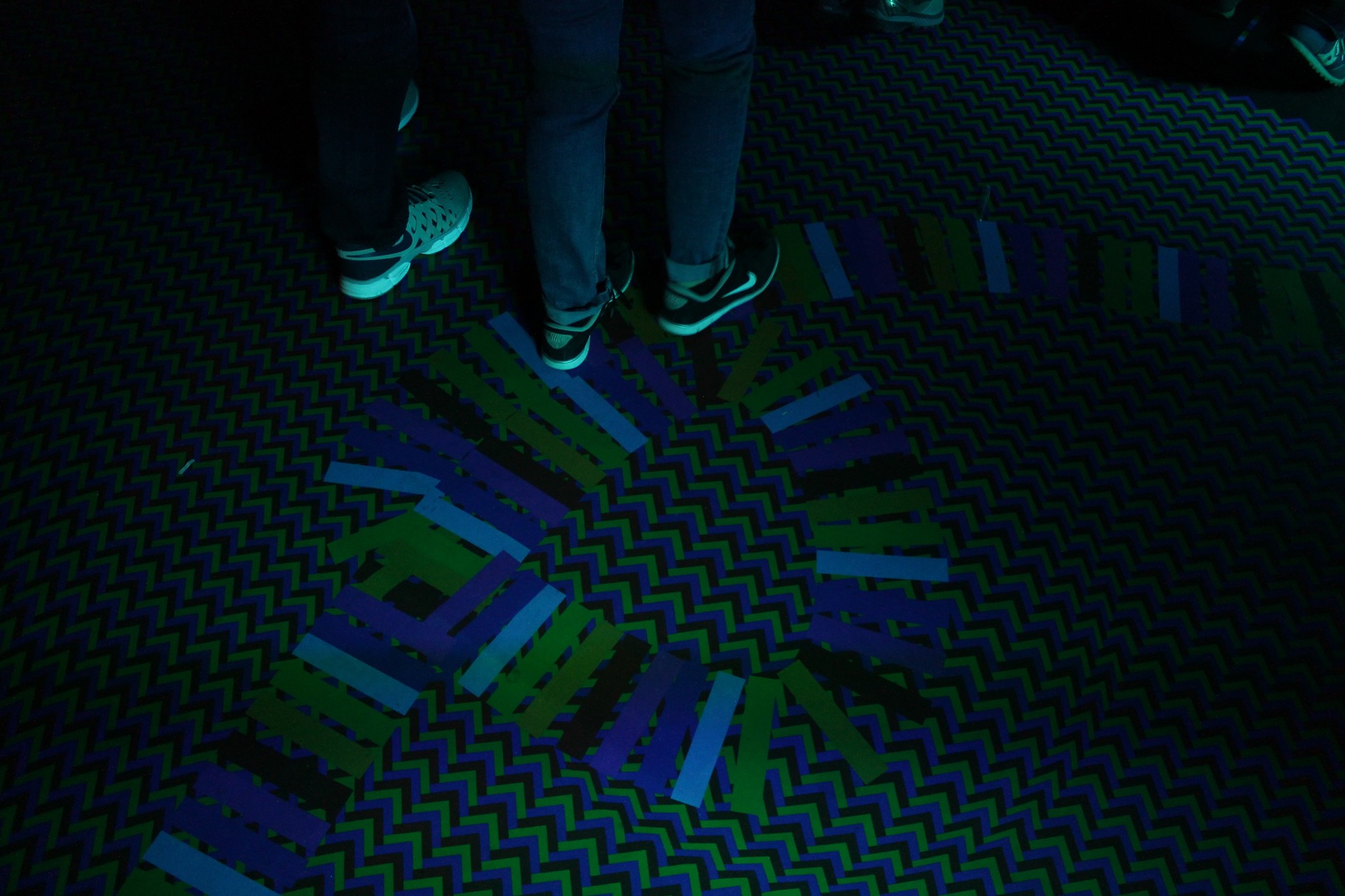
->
[
  {"xmin": 1287, "ymin": 24, "xmax": 1345, "ymax": 86},
  {"xmin": 859, "ymin": 0, "xmax": 945, "ymax": 32}
]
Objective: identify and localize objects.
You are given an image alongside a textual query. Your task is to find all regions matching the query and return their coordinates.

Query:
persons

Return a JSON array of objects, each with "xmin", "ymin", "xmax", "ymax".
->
[
  {"xmin": 311, "ymin": 0, "xmax": 474, "ymax": 300},
  {"xmin": 524, "ymin": 0, "xmax": 782, "ymax": 370},
  {"xmin": 1286, "ymin": 23, "xmax": 1345, "ymax": 87},
  {"xmin": 862, "ymin": 0, "xmax": 946, "ymax": 34}
]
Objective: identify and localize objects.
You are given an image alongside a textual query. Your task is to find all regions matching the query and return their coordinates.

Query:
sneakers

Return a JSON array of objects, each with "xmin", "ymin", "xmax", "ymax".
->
[
  {"xmin": 541, "ymin": 245, "xmax": 635, "ymax": 370},
  {"xmin": 334, "ymin": 171, "xmax": 472, "ymax": 300},
  {"xmin": 396, "ymin": 79, "xmax": 419, "ymax": 131},
  {"xmin": 657, "ymin": 229, "xmax": 780, "ymax": 336}
]
